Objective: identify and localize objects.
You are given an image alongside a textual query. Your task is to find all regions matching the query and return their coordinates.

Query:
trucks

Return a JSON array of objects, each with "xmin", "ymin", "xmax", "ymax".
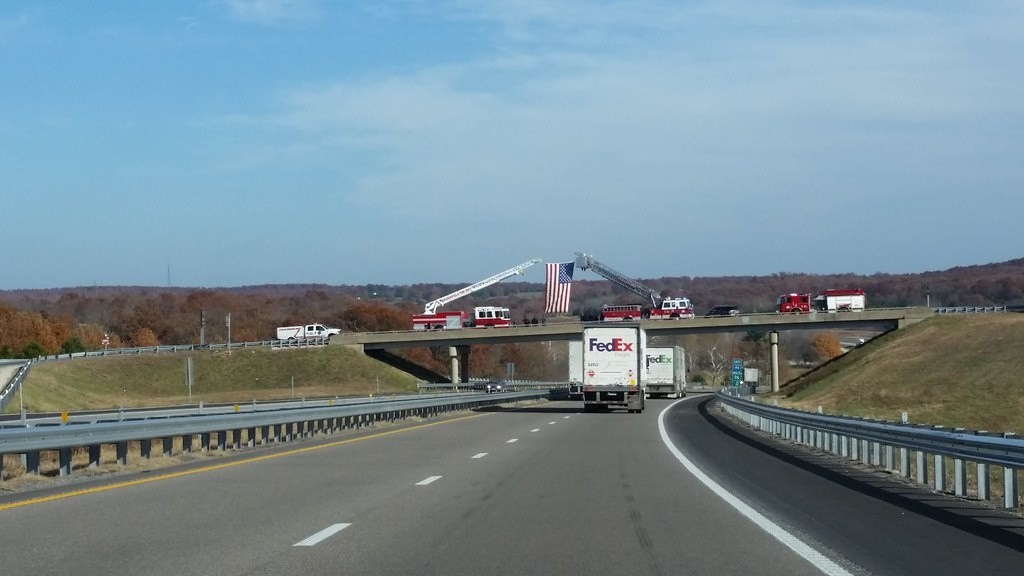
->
[
  {"xmin": 647, "ymin": 345, "xmax": 687, "ymax": 398},
  {"xmin": 582, "ymin": 324, "xmax": 650, "ymax": 413},
  {"xmin": 567, "ymin": 339, "xmax": 583, "ymax": 400}
]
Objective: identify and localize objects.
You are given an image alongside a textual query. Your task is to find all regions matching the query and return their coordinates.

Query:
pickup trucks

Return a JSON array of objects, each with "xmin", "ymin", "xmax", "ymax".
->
[{"xmin": 276, "ymin": 323, "xmax": 342, "ymax": 346}]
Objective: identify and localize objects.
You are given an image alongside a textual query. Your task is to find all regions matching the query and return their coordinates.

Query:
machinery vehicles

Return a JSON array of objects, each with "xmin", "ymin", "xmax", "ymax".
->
[
  {"xmin": 776, "ymin": 289, "xmax": 866, "ymax": 315},
  {"xmin": 574, "ymin": 250, "xmax": 696, "ymax": 322},
  {"xmin": 411, "ymin": 258, "xmax": 542, "ymax": 331}
]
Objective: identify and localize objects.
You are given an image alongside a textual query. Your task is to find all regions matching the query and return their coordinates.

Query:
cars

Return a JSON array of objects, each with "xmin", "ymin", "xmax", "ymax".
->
[
  {"xmin": 704, "ymin": 304, "xmax": 740, "ymax": 318},
  {"xmin": 484, "ymin": 379, "xmax": 505, "ymax": 392}
]
